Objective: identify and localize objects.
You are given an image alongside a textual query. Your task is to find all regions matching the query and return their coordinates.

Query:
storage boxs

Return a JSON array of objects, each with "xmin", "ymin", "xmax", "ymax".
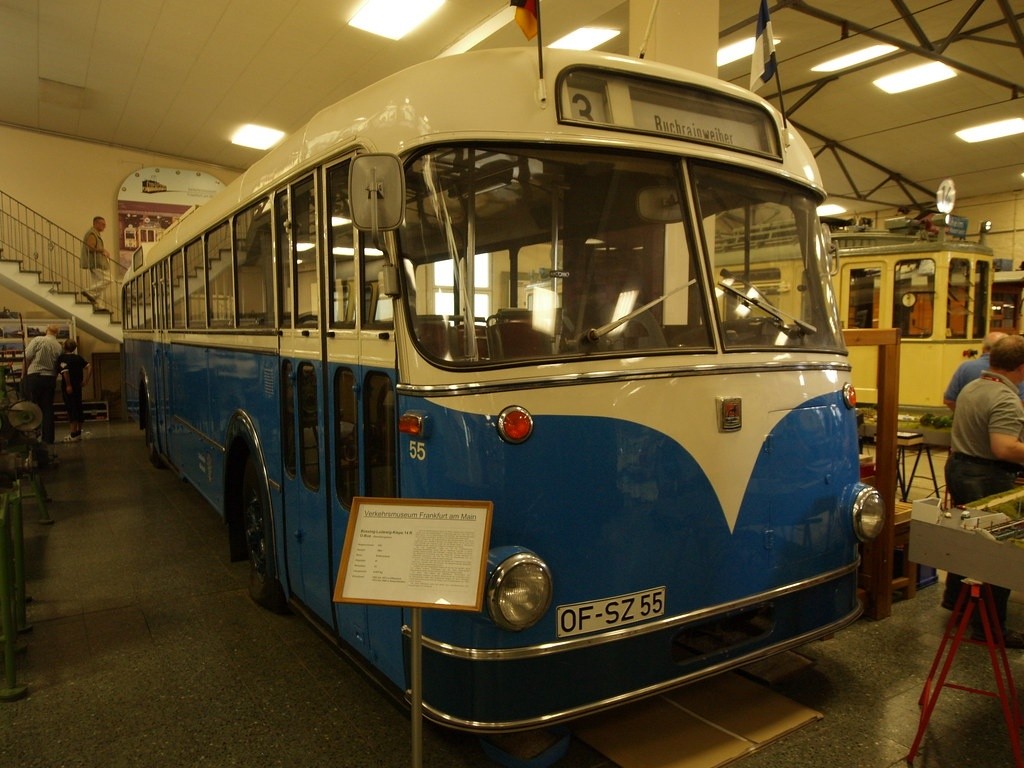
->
[{"xmin": 892, "ymin": 548, "xmax": 940, "ymax": 591}]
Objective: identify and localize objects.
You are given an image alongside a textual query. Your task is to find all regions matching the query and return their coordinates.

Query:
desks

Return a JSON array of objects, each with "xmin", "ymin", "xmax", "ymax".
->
[{"xmin": 862, "ymin": 435, "xmax": 940, "ymax": 504}]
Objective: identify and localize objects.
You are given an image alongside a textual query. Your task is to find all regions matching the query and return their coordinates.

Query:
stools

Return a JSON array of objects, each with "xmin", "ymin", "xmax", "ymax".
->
[{"xmin": 890, "ymin": 520, "xmax": 917, "ymax": 599}]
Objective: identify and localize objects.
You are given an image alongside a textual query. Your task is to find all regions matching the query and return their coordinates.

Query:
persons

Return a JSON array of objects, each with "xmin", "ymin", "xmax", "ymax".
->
[
  {"xmin": 79, "ymin": 215, "xmax": 112, "ymax": 309},
  {"xmin": 56, "ymin": 338, "xmax": 92, "ymax": 442},
  {"xmin": 941, "ymin": 331, "xmax": 1024, "ymax": 619},
  {"xmin": 20, "ymin": 323, "xmax": 63, "ymax": 444}
]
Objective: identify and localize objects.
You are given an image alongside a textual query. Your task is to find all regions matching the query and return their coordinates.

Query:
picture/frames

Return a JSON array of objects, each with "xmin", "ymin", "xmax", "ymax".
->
[{"xmin": 91, "ymin": 352, "xmax": 133, "ymax": 418}]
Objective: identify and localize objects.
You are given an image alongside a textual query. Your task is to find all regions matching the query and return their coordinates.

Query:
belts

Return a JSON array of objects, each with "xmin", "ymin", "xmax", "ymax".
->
[{"xmin": 950, "ymin": 451, "xmax": 1019, "ymax": 475}]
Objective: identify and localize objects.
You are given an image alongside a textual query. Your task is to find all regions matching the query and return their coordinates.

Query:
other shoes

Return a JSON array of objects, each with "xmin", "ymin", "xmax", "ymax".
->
[
  {"xmin": 81, "ymin": 291, "xmax": 95, "ymax": 302},
  {"xmin": 941, "ymin": 590, "xmax": 985, "ymax": 617},
  {"xmin": 60, "ymin": 433, "xmax": 82, "ymax": 443},
  {"xmin": 96, "ymin": 308, "xmax": 109, "ymax": 312},
  {"xmin": 971, "ymin": 628, "xmax": 1024, "ymax": 651}
]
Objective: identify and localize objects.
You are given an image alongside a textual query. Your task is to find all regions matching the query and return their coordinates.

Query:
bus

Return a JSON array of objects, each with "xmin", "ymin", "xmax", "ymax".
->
[
  {"xmin": 118, "ymin": 44, "xmax": 886, "ymax": 737},
  {"xmin": 510, "ymin": 204, "xmax": 997, "ymax": 420}
]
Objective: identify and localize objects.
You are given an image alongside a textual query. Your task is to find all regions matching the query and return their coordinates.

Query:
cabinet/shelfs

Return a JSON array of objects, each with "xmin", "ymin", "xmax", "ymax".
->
[
  {"xmin": 0, "ymin": 315, "xmax": 78, "ymax": 384},
  {"xmin": 54, "ymin": 401, "xmax": 110, "ymax": 424}
]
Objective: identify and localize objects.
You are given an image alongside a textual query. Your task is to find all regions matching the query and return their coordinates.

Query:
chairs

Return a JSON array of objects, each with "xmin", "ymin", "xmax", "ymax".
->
[
  {"xmin": 600, "ymin": 301, "xmax": 668, "ymax": 350},
  {"xmin": 417, "ymin": 314, "xmax": 449, "ymax": 359},
  {"xmin": 485, "ymin": 311, "xmax": 554, "ymax": 361},
  {"xmin": 446, "ymin": 316, "xmax": 489, "ymax": 361}
]
